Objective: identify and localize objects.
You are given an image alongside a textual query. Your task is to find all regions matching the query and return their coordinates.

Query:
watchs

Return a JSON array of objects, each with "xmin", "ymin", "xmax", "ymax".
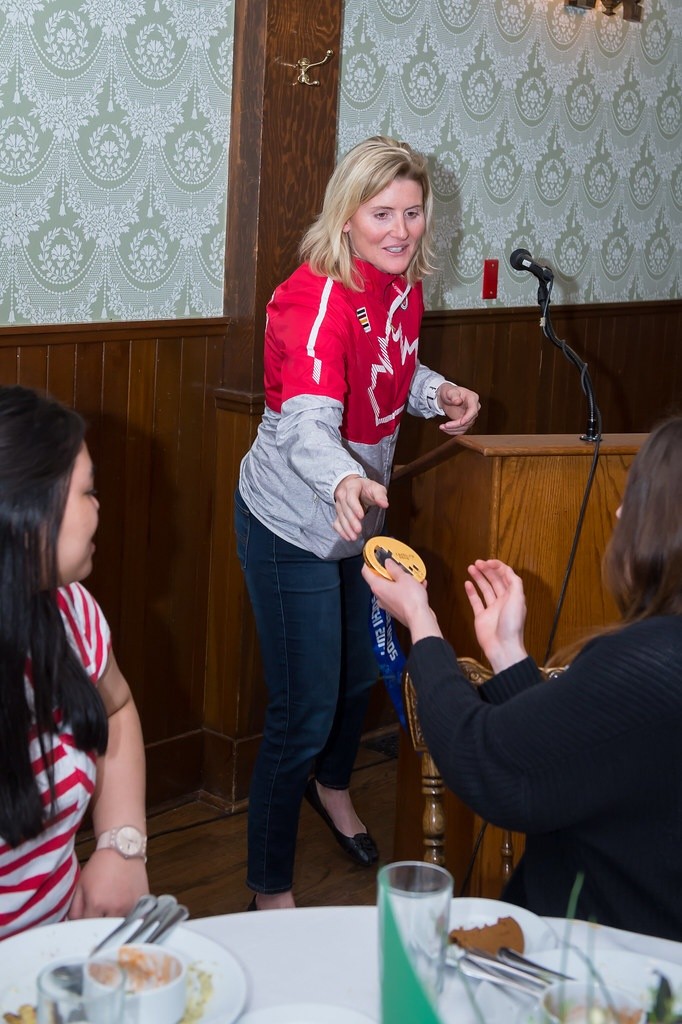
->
[{"xmin": 95, "ymin": 826, "xmax": 147, "ymax": 864}]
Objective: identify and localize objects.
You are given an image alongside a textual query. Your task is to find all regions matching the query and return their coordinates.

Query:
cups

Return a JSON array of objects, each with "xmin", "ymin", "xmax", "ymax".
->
[
  {"xmin": 83, "ymin": 943, "xmax": 186, "ymax": 1024},
  {"xmin": 36, "ymin": 960, "xmax": 125, "ymax": 1024},
  {"xmin": 377, "ymin": 862, "xmax": 452, "ymax": 997}
]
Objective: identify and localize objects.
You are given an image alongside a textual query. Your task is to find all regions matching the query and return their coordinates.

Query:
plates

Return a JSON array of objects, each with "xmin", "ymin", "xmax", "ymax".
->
[
  {"xmin": 234, "ymin": 1004, "xmax": 379, "ymax": 1024},
  {"xmin": 475, "ymin": 948, "xmax": 682, "ymax": 1024},
  {"xmin": 0, "ymin": 917, "xmax": 246, "ymax": 1024},
  {"xmin": 415, "ymin": 897, "xmax": 556, "ymax": 967}
]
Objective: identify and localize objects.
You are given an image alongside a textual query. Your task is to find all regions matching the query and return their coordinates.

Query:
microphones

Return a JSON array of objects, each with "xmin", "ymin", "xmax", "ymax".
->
[{"xmin": 509, "ymin": 249, "xmax": 553, "ymax": 281}]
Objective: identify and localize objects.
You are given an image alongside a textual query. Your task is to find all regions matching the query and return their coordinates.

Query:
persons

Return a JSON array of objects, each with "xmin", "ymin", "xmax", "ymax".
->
[
  {"xmin": 230, "ymin": 136, "xmax": 481, "ymax": 911},
  {"xmin": 362, "ymin": 414, "xmax": 682, "ymax": 945},
  {"xmin": 0, "ymin": 386, "xmax": 150, "ymax": 942}
]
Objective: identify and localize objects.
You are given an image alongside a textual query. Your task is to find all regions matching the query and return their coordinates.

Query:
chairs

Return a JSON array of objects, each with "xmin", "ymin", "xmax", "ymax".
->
[{"xmin": 404, "ymin": 658, "xmax": 569, "ymax": 895}]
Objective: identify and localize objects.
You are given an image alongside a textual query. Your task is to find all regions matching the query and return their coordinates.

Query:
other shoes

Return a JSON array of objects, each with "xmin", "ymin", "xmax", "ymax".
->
[
  {"xmin": 301, "ymin": 775, "xmax": 382, "ymax": 870},
  {"xmin": 246, "ymin": 892, "xmax": 259, "ymax": 912}
]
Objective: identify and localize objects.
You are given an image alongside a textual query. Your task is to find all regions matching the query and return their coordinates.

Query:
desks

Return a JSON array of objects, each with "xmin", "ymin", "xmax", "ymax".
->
[{"xmin": 182, "ymin": 904, "xmax": 682, "ymax": 1024}]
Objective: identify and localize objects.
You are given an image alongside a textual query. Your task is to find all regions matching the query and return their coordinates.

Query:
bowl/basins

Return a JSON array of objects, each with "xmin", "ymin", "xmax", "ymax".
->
[{"xmin": 541, "ymin": 983, "xmax": 647, "ymax": 1024}]
